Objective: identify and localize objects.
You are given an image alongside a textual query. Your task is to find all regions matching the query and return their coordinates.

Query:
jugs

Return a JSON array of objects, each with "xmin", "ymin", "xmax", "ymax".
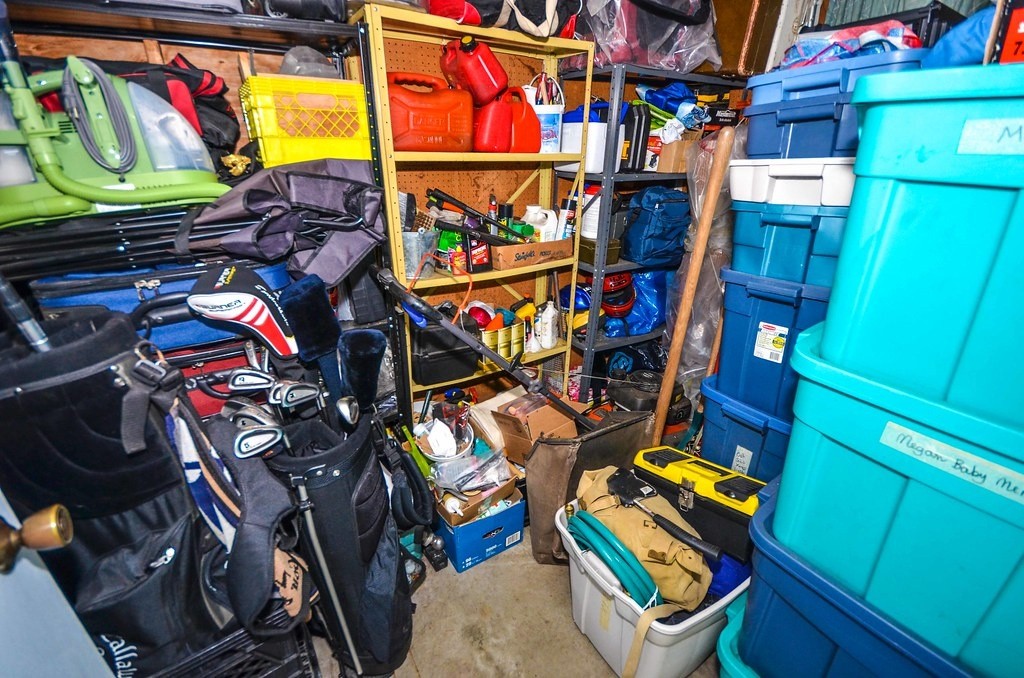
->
[
  {"xmin": 440, "ymin": 35, "xmax": 509, "ymax": 106},
  {"xmin": 470, "ymin": 87, "xmax": 541, "ymax": 153},
  {"xmin": 386, "ymin": 71, "xmax": 473, "ymax": 153}
]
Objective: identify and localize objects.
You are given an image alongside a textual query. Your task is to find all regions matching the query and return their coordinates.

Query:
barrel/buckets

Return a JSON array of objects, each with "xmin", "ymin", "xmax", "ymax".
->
[
  {"xmin": 521, "ymin": 72, "xmax": 565, "ymax": 153},
  {"xmin": 402, "ymin": 227, "xmax": 442, "ymax": 280},
  {"xmin": 415, "ymin": 419, "xmax": 474, "ymax": 463},
  {"xmin": 414, "ymin": 401, "xmax": 436, "ymax": 426}
]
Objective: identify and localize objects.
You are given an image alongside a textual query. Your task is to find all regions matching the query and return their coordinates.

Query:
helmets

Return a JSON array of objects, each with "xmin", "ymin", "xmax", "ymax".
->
[
  {"xmin": 586, "ymin": 270, "xmax": 636, "ymax": 315},
  {"xmin": 563, "ymin": 305, "xmax": 605, "ymax": 335},
  {"xmin": 556, "ymin": 282, "xmax": 591, "ymax": 310}
]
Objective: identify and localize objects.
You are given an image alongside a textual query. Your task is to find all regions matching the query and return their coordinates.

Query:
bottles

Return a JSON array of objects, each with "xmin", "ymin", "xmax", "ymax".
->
[
  {"xmin": 554, "ymin": 198, "xmax": 577, "ymax": 241},
  {"xmin": 510, "ymin": 293, "xmax": 559, "ymax": 352},
  {"xmin": 497, "ymin": 202, "xmax": 514, "ymax": 241}
]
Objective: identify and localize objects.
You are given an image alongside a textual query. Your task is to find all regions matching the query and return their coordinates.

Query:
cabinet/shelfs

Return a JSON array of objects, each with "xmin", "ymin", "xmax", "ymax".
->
[
  {"xmin": 0, "ymin": 0, "xmax": 407, "ymax": 427},
  {"xmin": 541, "ymin": 63, "xmax": 748, "ymax": 416},
  {"xmin": 346, "ymin": 3, "xmax": 595, "ymax": 429}
]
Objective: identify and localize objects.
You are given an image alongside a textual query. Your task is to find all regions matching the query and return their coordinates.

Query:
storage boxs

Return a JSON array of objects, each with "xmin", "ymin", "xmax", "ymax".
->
[
  {"xmin": 490, "ymin": 392, "xmax": 592, "ymax": 467},
  {"xmin": 554, "ymin": 469, "xmax": 752, "ymax": 678},
  {"xmin": 728, "ymin": 157, "xmax": 857, "ymax": 207},
  {"xmin": 818, "ymin": 62, "xmax": 1024, "ymax": 439},
  {"xmin": 148, "ymin": 605, "xmax": 324, "ymax": 678},
  {"xmin": 401, "ymin": 415, "xmax": 519, "ymax": 527},
  {"xmin": 772, "ymin": 320, "xmax": 1024, "ymax": 678},
  {"xmin": 799, "ymin": 0, "xmax": 971, "ymax": 48},
  {"xmin": 238, "ymin": 76, "xmax": 373, "ymax": 169},
  {"xmin": 729, "ymin": 200, "xmax": 850, "ymax": 288},
  {"xmin": 489, "ymin": 235, "xmax": 573, "ymax": 272},
  {"xmin": 431, "ymin": 498, "xmax": 526, "ymax": 574},
  {"xmin": 468, "ymin": 415, "xmax": 526, "ymax": 484},
  {"xmin": 515, "ymin": 483, "xmax": 530, "ymax": 527},
  {"xmin": 738, "ymin": 469, "xmax": 982, "ymax": 678},
  {"xmin": 714, "ymin": 265, "xmax": 832, "ymax": 425},
  {"xmin": 743, "ymin": 91, "xmax": 859, "ymax": 159},
  {"xmin": 571, "ymin": 232, "xmax": 621, "ymax": 266},
  {"xmin": 715, "ymin": 590, "xmax": 764, "ymax": 678},
  {"xmin": 640, "ymin": 127, "xmax": 703, "ymax": 173},
  {"xmin": 692, "ymin": 0, "xmax": 783, "ymax": 80},
  {"xmin": 633, "ymin": 445, "xmax": 768, "ymax": 568},
  {"xmin": 693, "ymin": 106, "xmax": 741, "ymax": 131},
  {"xmin": 700, "ymin": 374, "xmax": 794, "ymax": 484},
  {"xmin": 422, "ymin": 543, "xmax": 448, "ymax": 572},
  {"xmin": 746, "ymin": 47, "xmax": 934, "ymax": 107},
  {"xmin": 453, "ymin": 302, "xmax": 526, "ymax": 374},
  {"xmin": 685, "ymin": 84, "xmax": 729, "ymax": 110}
]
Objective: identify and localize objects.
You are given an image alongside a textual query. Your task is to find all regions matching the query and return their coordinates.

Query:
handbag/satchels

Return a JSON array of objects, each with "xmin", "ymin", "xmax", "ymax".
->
[
  {"xmin": 470, "ymin": 0, "xmax": 590, "ymax": 38},
  {"xmin": 577, "ymin": 467, "xmax": 713, "ymax": 614},
  {"xmin": 619, "ymin": 185, "xmax": 692, "ymax": 266}
]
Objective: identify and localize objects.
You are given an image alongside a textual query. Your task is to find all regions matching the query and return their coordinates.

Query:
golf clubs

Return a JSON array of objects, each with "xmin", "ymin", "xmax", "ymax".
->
[{"xmin": 185, "ymin": 263, "xmax": 387, "ymax": 461}]
